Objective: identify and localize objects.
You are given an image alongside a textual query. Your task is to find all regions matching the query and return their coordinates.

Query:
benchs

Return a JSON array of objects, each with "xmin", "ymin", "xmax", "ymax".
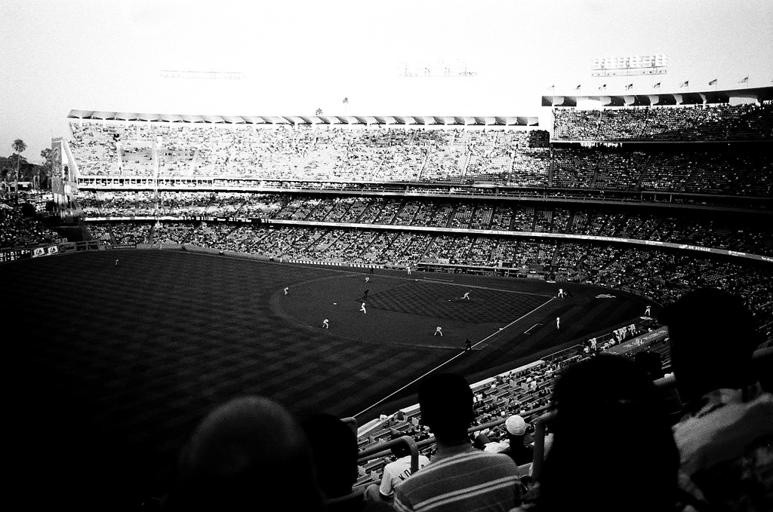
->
[{"xmin": 351, "ymin": 355, "xmax": 592, "ymax": 490}]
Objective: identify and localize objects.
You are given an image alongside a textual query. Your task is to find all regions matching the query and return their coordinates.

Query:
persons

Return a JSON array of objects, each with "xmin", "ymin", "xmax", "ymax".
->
[
  {"xmin": 322, "ymin": 317, "xmax": 329, "ymax": 330},
  {"xmin": 276, "ymin": 406, "xmax": 359, "ymax": 501},
  {"xmin": 171, "ymin": 393, "xmax": 316, "ymax": 509},
  {"xmin": 352, "ymin": 265, "xmax": 772, "ymax": 512},
  {"xmin": 0, "ymin": 101, "xmax": 770, "ymax": 264}
]
